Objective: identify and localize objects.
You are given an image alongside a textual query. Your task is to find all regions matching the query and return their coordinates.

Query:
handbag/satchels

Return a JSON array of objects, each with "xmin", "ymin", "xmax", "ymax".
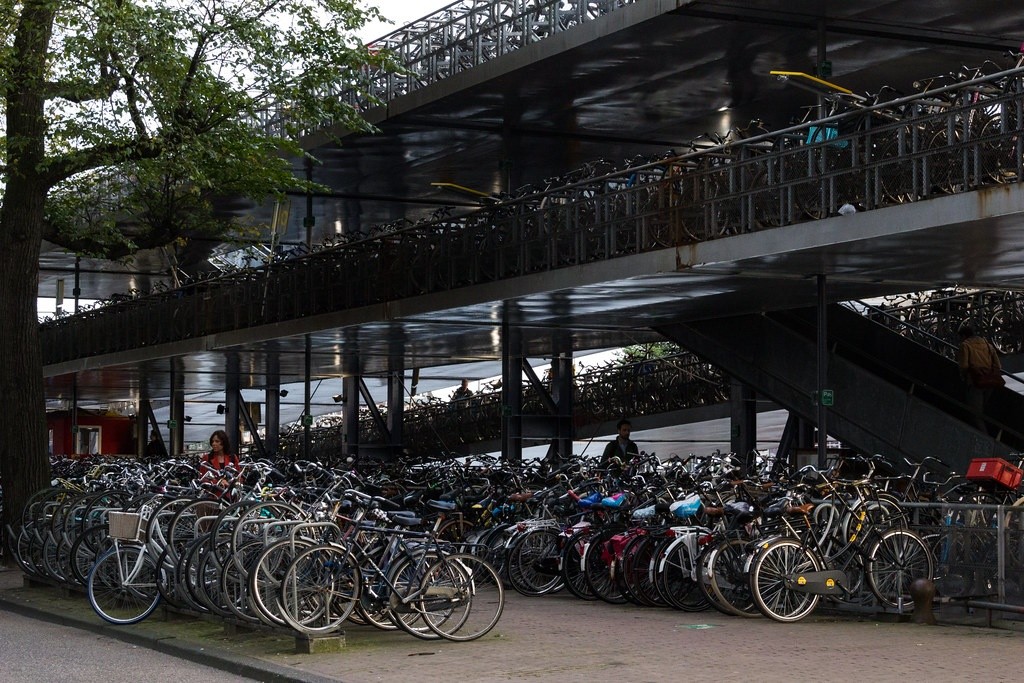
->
[{"xmin": 987, "ymin": 338, "xmax": 1006, "ymax": 388}]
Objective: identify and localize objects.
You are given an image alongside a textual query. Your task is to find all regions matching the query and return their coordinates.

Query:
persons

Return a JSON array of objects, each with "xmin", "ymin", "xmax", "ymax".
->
[
  {"xmin": 958, "ymin": 327, "xmax": 1002, "ymax": 434},
  {"xmin": 598, "ymin": 419, "xmax": 638, "ymax": 476},
  {"xmin": 451, "ymin": 378, "xmax": 473, "ymax": 401},
  {"xmin": 198, "ymin": 430, "xmax": 240, "ymax": 506},
  {"xmin": 145, "ymin": 429, "xmax": 170, "ymax": 459}
]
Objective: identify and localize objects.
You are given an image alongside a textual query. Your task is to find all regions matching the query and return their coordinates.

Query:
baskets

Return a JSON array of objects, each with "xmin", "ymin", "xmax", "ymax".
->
[
  {"xmin": 966, "ymin": 457, "xmax": 1024, "ymax": 490},
  {"xmin": 108, "ymin": 510, "xmax": 140, "ymax": 538},
  {"xmin": 806, "ymin": 126, "xmax": 848, "ymax": 150},
  {"xmin": 196, "ymin": 503, "xmax": 221, "ymax": 531}
]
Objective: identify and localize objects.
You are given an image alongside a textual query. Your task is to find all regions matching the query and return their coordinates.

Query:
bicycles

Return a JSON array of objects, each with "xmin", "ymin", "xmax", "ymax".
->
[{"xmin": 0, "ymin": 0, "xmax": 1024, "ymax": 642}]
